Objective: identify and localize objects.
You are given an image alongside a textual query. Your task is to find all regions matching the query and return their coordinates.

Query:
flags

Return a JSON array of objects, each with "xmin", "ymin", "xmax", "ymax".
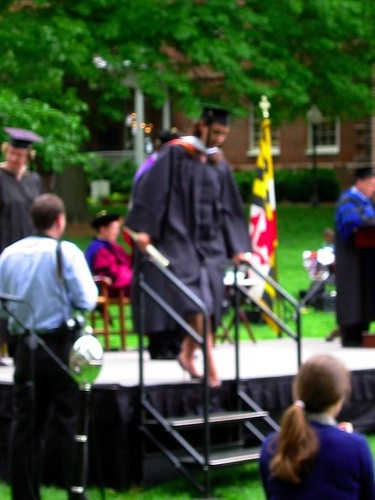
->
[{"xmin": 247, "ymin": 118, "xmax": 280, "ymax": 332}]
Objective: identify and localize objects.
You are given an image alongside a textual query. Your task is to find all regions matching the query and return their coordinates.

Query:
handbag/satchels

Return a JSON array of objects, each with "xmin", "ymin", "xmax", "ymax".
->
[{"xmin": 63, "ymin": 313, "xmax": 87, "ymax": 353}]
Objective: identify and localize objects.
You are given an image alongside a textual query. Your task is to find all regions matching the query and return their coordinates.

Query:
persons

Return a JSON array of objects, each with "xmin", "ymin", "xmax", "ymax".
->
[
  {"xmin": 259, "ymin": 353, "xmax": 375, "ymax": 500},
  {"xmin": 126, "ymin": 103, "xmax": 254, "ymax": 388},
  {"xmin": 85, "ymin": 209, "xmax": 133, "ymax": 297},
  {"xmin": 334, "ymin": 167, "xmax": 375, "ymax": 347},
  {"xmin": 0, "ymin": 192, "xmax": 98, "ymax": 500},
  {"xmin": 0, "ymin": 127, "xmax": 43, "ymax": 365}
]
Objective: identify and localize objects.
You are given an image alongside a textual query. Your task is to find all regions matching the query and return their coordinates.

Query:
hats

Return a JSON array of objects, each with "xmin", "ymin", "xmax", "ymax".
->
[
  {"xmin": 199, "ymin": 106, "xmax": 237, "ymax": 126},
  {"xmin": 352, "ymin": 166, "xmax": 375, "ymax": 179},
  {"xmin": 3, "ymin": 127, "xmax": 42, "ymax": 148}
]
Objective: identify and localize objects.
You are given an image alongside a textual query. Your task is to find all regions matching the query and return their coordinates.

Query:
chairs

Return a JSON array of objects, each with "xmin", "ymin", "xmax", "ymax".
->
[
  {"xmin": 90, "ymin": 275, "xmax": 132, "ymax": 351},
  {"xmin": 213, "ymin": 296, "xmax": 257, "ymax": 348}
]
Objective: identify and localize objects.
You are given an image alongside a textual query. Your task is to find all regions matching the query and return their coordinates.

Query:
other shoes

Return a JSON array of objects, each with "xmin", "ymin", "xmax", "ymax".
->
[
  {"xmin": 177, "ymin": 355, "xmax": 202, "ymax": 380},
  {"xmin": 208, "ymin": 379, "xmax": 222, "ymax": 389}
]
